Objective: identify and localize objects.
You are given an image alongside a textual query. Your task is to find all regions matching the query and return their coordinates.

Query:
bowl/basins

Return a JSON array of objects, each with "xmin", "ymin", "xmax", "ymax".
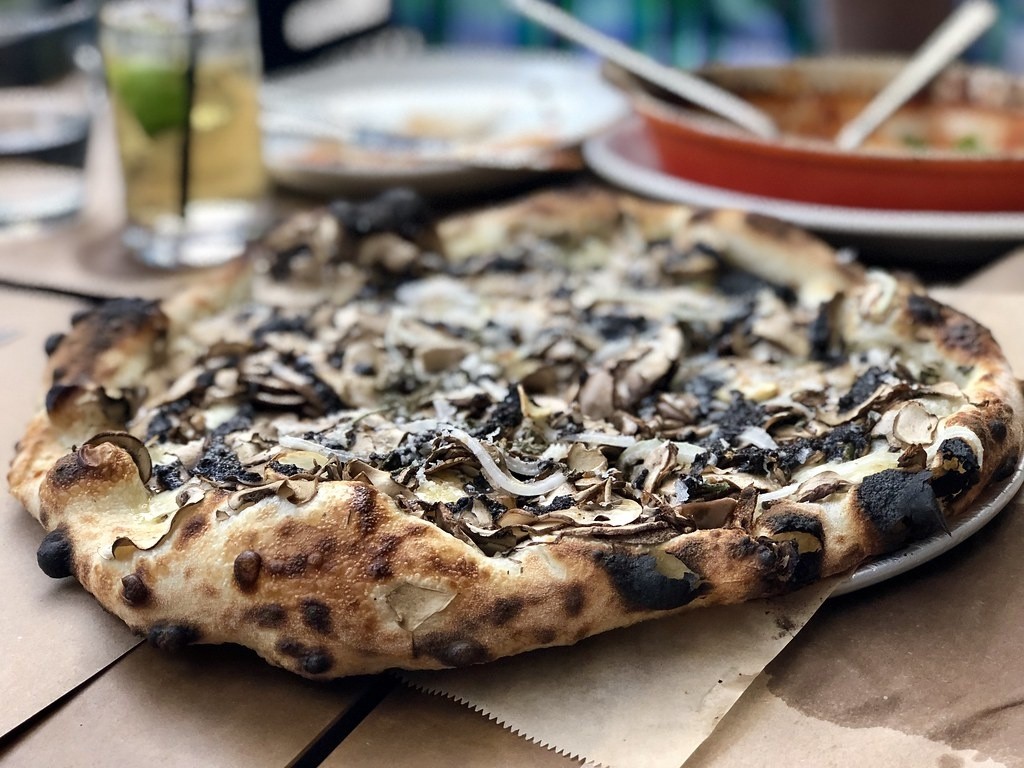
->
[
  {"xmin": 604, "ymin": 58, "xmax": 1024, "ymax": 214},
  {"xmin": 583, "ymin": 119, "xmax": 1024, "ymax": 287}
]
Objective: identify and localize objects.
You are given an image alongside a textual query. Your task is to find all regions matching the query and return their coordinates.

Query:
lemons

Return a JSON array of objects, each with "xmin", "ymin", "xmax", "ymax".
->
[{"xmin": 114, "ymin": 67, "xmax": 185, "ymax": 133}]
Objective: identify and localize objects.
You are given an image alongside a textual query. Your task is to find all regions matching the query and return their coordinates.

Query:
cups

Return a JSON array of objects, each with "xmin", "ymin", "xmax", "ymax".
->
[
  {"xmin": 98, "ymin": 1, "xmax": 273, "ymax": 270},
  {"xmin": 0, "ymin": 0, "xmax": 99, "ymax": 226}
]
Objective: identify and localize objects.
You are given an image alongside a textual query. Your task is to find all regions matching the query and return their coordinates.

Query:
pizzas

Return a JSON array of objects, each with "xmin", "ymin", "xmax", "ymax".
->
[{"xmin": 9, "ymin": 190, "xmax": 1024, "ymax": 684}]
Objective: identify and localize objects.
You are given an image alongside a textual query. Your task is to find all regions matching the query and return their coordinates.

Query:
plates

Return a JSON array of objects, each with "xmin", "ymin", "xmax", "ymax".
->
[
  {"xmin": 266, "ymin": 49, "xmax": 585, "ymax": 203},
  {"xmin": 830, "ymin": 452, "xmax": 1024, "ymax": 598}
]
